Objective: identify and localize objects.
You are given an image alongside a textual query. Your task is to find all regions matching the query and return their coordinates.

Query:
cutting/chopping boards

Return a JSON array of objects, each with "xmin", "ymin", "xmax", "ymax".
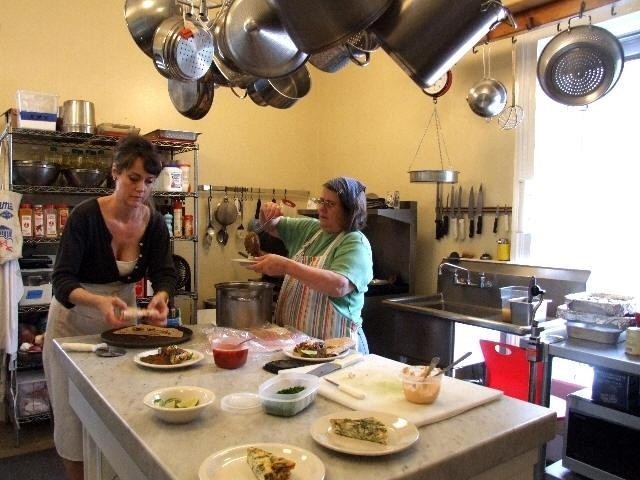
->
[{"xmin": 279, "ymin": 353, "xmax": 505, "ymax": 427}]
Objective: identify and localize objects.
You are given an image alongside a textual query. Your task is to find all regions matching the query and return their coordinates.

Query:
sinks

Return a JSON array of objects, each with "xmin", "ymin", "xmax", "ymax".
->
[{"xmin": 386, "ymin": 294, "xmax": 502, "ymax": 319}]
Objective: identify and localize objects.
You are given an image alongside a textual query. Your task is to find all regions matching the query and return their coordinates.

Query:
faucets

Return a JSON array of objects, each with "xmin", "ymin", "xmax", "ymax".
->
[{"xmin": 438, "ymin": 262, "xmax": 471, "ymax": 285}]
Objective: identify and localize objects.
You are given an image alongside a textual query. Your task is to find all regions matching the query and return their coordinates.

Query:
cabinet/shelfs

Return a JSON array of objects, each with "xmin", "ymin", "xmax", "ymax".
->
[
  {"xmin": 0, "ymin": 124, "xmax": 202, "ymax": 448},
  {"xmin": 297, "ymin": 200, "xmax": 419, "ymax": 298}
]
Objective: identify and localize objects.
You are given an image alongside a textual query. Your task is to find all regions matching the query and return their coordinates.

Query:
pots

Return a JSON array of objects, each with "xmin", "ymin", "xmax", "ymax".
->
[
  {"xmin": 124, "ymin": 0, "xmax": 518, "ymax": 122},
  {"xmin": 212, "ymin": 280, "xmax": 276, "ymax": 332}
]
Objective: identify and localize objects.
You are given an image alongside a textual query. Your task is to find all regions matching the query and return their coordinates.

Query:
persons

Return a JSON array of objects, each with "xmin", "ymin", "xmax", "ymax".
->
[
  {"xmin": 240, "ymin": 176, "xmax": 374, "ymax": 355},
  {"xmin": 41, "ymin": 135, "xmax": 176, "ymax": 480}
]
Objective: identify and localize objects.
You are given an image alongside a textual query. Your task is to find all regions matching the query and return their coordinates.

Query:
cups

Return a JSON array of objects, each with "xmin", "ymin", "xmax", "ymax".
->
[
  {"xmin": 211, "ymin": 337, "xmax": 249, "ymax": 370},
  {"xmin": 403, "ymin": 365, "xmax": 441, "ymax": 404}
]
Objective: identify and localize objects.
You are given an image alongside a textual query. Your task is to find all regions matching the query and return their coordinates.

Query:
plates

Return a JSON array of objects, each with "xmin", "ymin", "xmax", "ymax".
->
[
  {"xmin": 310, "ymin": 410, "xmax": 420, "ymax": 457},
  {"xmin": 199, "ymin": 442, "xmax": 325, "ymax": 480},
  {"xmin": 231, "ymin": 257, "xmax": 261, "ymax": 266},
  {"xmin": 282, "ymin": 345, "xmax": 350, "ymax": 361},
  {"xmin": 134, "ymin": 349, "xmax": 205, "ymax": 369}
]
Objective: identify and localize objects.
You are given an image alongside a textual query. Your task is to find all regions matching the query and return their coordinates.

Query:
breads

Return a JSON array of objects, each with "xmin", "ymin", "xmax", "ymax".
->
[
  {"xmin": 247, "ymin": 446, "xmax": 296, "ymax": 480},
  {"xmin": 330, "ymin": 416, "xmax": 386, "ymax": 443}
]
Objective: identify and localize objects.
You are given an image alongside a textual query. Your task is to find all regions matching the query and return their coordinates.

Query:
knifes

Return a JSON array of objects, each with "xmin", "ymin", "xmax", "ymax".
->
[
  {"xmin": 304, "ymin": 357, "xmax": 362, "ymax": 378},
  {"xmin": 433, "ymin": 182, "xmax": 483, "ymax": 241},
  {"xmin": 492, "ymin": 205, "xmax": 500, "ymax": 233},
  {"xmin": 503, "ymin": 205, "xmax": 509, "ymax": 231}
]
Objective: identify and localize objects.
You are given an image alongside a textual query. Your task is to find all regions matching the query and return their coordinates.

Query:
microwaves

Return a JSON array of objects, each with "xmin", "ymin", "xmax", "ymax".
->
[{"xmin": 563, "ymin": 383, "xmax": 639, "ymax": 480}]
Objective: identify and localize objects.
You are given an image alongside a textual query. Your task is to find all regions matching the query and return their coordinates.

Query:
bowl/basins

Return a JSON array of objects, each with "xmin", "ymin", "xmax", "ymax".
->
[
  {"xmin": 58, "ymin": 99, "xmax": 96, "ymax": 136},
  {"xmin": 144, "ymin": 386, "xmax": 216, "ymax": 424},
  {"xmin": 12, "ymin": 159, "xmax": 61, "ymax": 186},
  {"xmin": 63, "ymin": 166, "xmax": 109, "ymax": 188}
]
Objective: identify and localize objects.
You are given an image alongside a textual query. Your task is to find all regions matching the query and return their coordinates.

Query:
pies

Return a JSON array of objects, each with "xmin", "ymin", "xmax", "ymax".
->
[
  {"xmin": 113, "ymin": 322, "xmax": 185, "ymax": 338},
  {"xmin": 324, "ymin": 337, "xmax": 357, "ymax": 357},
  {"xmin": 119, "ymin": 307, "xmax": 159, "ymax": 318}
]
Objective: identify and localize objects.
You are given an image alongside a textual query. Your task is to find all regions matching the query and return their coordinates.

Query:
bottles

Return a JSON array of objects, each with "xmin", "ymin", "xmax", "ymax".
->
[
  {"xmin": 496, "ymin": 239, "xmax": 510, "ymax": 262},
  {"xmin": 29, "ymin": 144, "xmax": 107, "ymax": 166},
  {"xmin": 159, "ymin": 162, "xmax": 191, "ymax": 194},
  {"xmin": 17, "ymin": 203, "xmax": 74, "ymax": 240},
  {"xmin": 165, "ymin": 199, "xmax": 192, "ymax": 238}
]
